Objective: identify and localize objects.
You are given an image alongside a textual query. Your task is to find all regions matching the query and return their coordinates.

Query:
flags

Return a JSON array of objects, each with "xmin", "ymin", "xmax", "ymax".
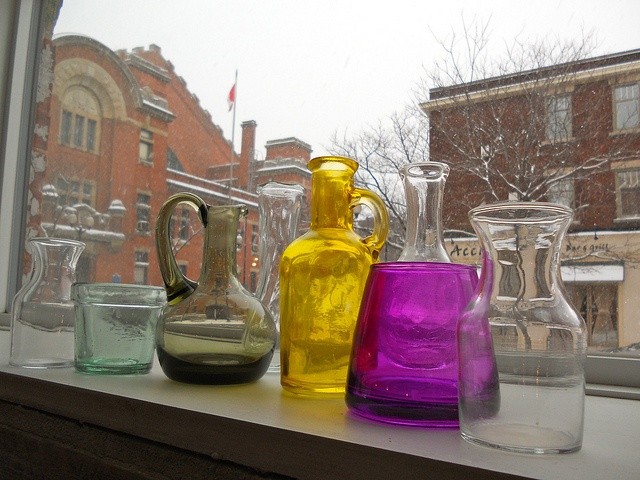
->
[{"xmin": 225, "ymin": 81, "xmax": 236, "ymax": 112}]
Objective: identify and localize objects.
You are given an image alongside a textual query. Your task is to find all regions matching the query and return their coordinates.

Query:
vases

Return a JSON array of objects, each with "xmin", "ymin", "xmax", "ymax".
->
[
  {"xmin": 253, "ymin": 181, "xmax": 306, "ymax": 376},
  {"xmin": 457, "ymin": 203, "xmax": 587, "ymax": 452},
  {"xmin": 373, "ymin": 160, "xmax": 458, "ymax": 369},
  {"xmin": 9, "ymin": 236, "xmax": 87, "ymax": 372}
]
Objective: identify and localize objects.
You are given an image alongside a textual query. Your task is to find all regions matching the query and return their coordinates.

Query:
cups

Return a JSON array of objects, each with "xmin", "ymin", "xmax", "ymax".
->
[{"xmin": 70, "ymin": 282, "xmax": 167, "ymax": 375}]
